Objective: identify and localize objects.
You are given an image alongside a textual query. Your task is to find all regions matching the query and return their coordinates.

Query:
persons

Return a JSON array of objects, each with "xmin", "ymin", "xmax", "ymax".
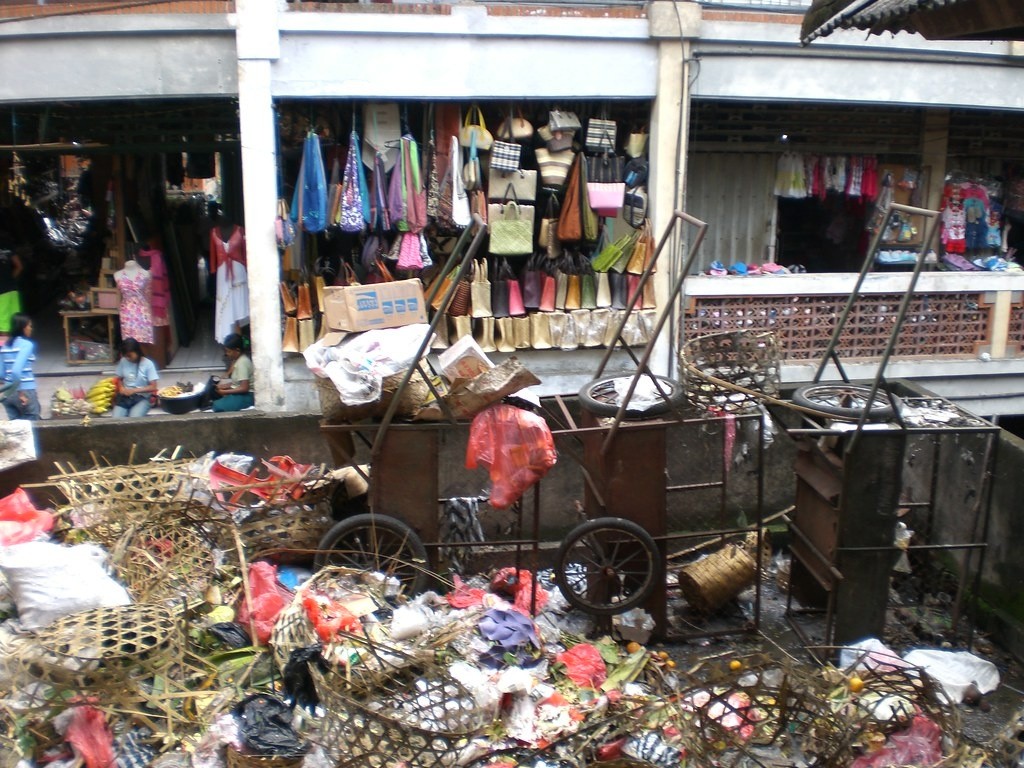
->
[
  {"xmin": 1000, "ymin": 195, "xmax": 1024, "ymax": 268},
  {"xmin": 212, "ymin": 333, "xmax": 254, "ymax": 412},
  {"xmin": 125, "ymin": 244, "xmax": 171, "ymax": 327},
  {"xmin": 117, "ymin": 338, "xmax": 157, "ymax": 418},
  {"xmin": 113, "ymin": 261, "xmax": 155, "ymax": 344},
  {"xmin": 0, "ymin": 312, "xmax": 42, "ymax": 420},
  {"xmin": 195, "ymin": 202, "xmax": 225, "ymax": 306},
  {"xmin": 209, "ymin": 204, "xmax": 250, "ymax": 345},
  {"xmin": 826, "ymin": 201, "xmax": 857, "ymax": 272}
]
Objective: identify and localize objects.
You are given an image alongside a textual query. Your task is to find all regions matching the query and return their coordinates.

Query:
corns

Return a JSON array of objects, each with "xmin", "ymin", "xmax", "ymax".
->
[{"xmin": 85, "ymin": 377, "xmax": 116, "ymax": 413}]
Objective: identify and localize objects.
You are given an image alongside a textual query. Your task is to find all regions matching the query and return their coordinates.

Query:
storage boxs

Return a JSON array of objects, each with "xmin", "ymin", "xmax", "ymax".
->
[
  {"xmin": 314, "ymin": 364, "xmax": 447, "ymax": 423},
  {"xmin": 322, "ymin": 278, "xmax": 428, "ymax": 347}
]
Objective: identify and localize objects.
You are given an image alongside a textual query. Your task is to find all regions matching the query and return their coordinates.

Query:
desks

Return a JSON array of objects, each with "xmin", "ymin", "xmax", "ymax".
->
[
  {"xmin": 59, "ymin": 311, "xmax": 118, "ymax": 364},
  {"xmin": 879, "ymin": 261, "xmax": 937, "ymax": 272}
]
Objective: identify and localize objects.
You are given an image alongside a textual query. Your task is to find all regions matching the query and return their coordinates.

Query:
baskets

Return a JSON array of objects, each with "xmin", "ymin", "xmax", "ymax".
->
[
  {"xmin": 746, "ymin": 526, "xmax": 772, "ymax": 575},
  {"xmin": 678, "ymin": 543, "xmax": 756, "ymax": 614},
  {"xmin": 776, "ymin": 559, "xmax": 792, "ymax": 593}
]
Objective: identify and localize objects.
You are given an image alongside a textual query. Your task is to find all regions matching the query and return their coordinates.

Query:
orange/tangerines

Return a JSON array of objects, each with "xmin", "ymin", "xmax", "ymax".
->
[{"xmin": 628, "ymin": 641, "xmax": 863, "ymax": 704}]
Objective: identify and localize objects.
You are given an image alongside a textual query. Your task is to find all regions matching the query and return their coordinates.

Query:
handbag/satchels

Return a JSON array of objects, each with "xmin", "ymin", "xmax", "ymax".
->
[
  {"xmin": 276, "ymin": 103, "xmax": 660, "ymax": 353},
  {"xmin": 159, "ymin": 172, "xmax": 186, "ymax": 206},
  {"xmin": 181, "ymin": 173, "xmax": 225, "ymax": 219}
]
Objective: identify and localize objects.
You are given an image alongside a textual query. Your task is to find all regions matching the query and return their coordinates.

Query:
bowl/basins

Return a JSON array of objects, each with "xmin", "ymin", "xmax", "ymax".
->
[{"xmin": 159, "ymin": 392, "xmax": 204, "ymax": 413}]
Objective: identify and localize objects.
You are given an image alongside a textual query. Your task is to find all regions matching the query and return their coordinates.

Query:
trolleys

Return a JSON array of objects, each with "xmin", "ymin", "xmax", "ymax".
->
[
  {"xmin": 540, "ymin": 211, "xmax": 768, "ymax": 640},
  {"xmin": 783, "ymin": 202, "xmax": 1001, "ymax": 667},
  {"xmin": 315, "ymin": 213, "xmax": 551, "ymax": 622}
]
